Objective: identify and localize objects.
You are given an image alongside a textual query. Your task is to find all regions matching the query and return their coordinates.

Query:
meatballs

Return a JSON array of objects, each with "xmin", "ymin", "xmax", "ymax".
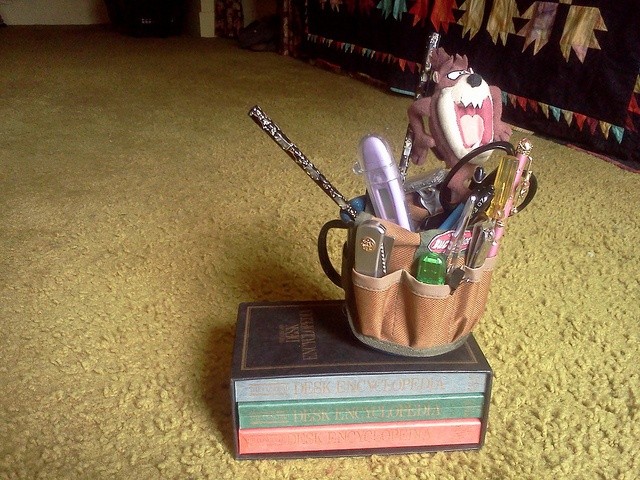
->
[{"xmin": 486, "ymin": 155, "xmax": 520, "ymax": 228}]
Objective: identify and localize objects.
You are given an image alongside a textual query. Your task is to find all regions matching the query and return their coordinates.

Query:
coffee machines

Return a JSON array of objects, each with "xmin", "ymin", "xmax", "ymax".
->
[{"xmin": 414, "ymin": 140, "xmax": 538, "ymax": 235}]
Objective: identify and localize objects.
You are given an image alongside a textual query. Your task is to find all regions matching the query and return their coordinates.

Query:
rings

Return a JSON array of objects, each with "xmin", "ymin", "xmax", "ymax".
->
[
  {"xmin": 487, "ymin": 137, "xmax": 535, "ymax": 257},
  {"xmin": 450, "ymin": 204, "xmax": 474, "ymax": 270},
  {"xmin": 467, "ymin": 186, "xmax": 496, "ymax": 226},
  {"xmin": 438, "ymin": 187, "xmax": 482, "ymax": 230},
  {"xmin": 399, "ymin": 32, "xmax": 442, "ymax": 184},
  {"xmin": 444, "ymin": 195, "xmax": 477, "ymax": 261},
  {"xmin": 249, "ymin": 106, "xmax": 359, "ymax": 222}
]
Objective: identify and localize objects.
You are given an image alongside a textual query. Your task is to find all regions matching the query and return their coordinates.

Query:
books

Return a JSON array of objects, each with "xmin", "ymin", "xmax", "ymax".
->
[
  {"xmin": 238, "ymin": 396, "xmax": 484, "ymax": 429},
  {"xmin": 240, "ymin": 417, "xmax": 481, "ymax": 454},
  {"xmin": 231, "ymin": 303, "xmax": 487, "ymax": 402}
]
